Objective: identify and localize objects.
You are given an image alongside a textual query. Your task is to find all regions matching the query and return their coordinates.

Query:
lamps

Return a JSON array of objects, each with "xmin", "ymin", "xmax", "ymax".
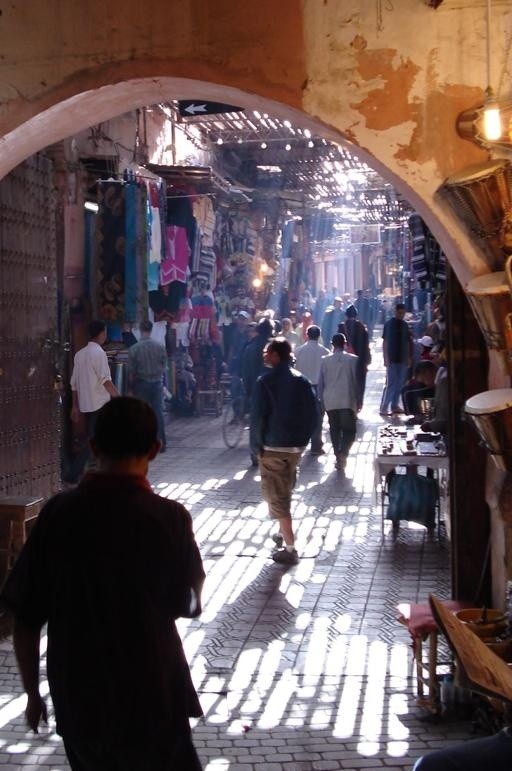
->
[{"xmin": 481, "ymin": 1, "xmax": 502, "ymax": 142}]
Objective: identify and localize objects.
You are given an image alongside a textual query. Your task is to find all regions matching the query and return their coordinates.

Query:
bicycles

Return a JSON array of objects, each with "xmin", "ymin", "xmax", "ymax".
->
[{"xmin": 222, "ymin": 370, "xmax": 253, "ymax": 452}]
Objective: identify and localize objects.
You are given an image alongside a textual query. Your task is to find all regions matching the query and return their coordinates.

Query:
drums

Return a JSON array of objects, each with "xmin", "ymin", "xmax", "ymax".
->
[
  {"xmin": 465, "ymin": 388, "xmax": 512, "ymax": 473},
  {"xmin": 466, "ymin": 269, "xmax": 512, "ymax": 375},
  {"xmin": 446, "ymin": 159, "xmax": 512, "ymax": 265}
]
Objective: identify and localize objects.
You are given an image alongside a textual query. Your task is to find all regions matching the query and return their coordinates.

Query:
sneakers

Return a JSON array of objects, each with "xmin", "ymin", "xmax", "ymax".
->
[
  {"xmin": 379, "ymin": 406, "xmax": 404, "ymax": 416},
  {"xmin": 273, "ymin": 533, "xmax": 283, "ymax": 548},
  {"xmin": 336, "ymin": 452, "xmax": 347, "ymax": 469},
  {"xmin": 310, "ymin": 448, "xmax": 325, "ymax": 455},
  {"xmin": 403, "ymin": 418, "xmax": 423, "ymax": 425},
  {"xmin": 273, "ymin": 547, "xmax": 298, "ymax": 564}
]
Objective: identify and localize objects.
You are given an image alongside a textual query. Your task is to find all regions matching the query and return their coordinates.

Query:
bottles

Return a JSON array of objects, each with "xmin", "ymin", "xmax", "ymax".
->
[{"xmin": 429, "ymin": 673, "xmax": 462, "ymax": 715}]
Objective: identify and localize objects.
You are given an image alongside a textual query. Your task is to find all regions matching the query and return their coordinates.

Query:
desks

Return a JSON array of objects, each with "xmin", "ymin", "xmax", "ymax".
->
[{"xmin": 375, "ymin": 424, "xmax": 448, "ymax": 542}]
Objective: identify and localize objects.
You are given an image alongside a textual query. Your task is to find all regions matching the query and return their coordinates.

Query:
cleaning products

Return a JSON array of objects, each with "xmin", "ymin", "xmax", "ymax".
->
[{"xmin": 439, "ymin": 673, "xmax": 454, "ymax": 703}]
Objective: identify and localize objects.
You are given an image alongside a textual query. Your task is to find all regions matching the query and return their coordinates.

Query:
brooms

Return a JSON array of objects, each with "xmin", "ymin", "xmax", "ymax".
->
[{"xmin": 461, "ymin": 540, "xmax": 492, "ymax": 607}]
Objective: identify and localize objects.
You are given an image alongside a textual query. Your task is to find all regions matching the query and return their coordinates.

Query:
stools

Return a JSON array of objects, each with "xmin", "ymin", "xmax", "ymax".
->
[
  {"xmin": 396, "ymin": 600, "xmax": 478, "ymax": 710},
  {"xmin": 1, "ymin": 495, "xmax": 45, "ymax": 585}
]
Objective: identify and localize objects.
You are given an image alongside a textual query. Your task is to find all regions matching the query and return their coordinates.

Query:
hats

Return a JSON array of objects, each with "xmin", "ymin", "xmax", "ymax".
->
[
  {"xmin": 417, "ymin": 335, "xmax": 434, "ymax": 348},
  {"xmin": 329, "ymin": 333, "xmax": 347, "ymax": 344},
  {"xmin": 345, "ymin": 304, "xmax": 358, "ymax": 316}
]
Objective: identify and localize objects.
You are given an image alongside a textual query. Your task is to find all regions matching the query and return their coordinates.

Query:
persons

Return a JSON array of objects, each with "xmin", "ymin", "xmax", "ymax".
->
[
  {"xmin": 3, "ymin": 396, "xmax": 204, "ymax": 771},
  {"xmin": 71, "ymin": 318, "xmax": 119, "ymax": 440},
  {"xmin": 172, "ymin": 284, "xmax": 443, "ymax": 473},
  {"xmin": 127, "ymin": 319, "xmax": 168, "ymax": 455},
  {"xmin": 248, "ymin": 335, "xmax": 320, "ymax": 567}
]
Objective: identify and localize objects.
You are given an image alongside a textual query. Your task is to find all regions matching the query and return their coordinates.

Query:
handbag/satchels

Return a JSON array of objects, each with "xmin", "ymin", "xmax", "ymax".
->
[{"xmin": 382, "ymin": 473, "xmax": 438, "ymax": 529}]
